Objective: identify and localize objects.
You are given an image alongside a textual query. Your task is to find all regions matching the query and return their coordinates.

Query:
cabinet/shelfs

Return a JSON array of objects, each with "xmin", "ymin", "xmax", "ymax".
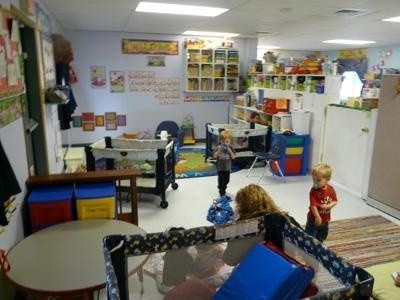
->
[
  {"xmin": 184, "ymin": 48, "xmax": 240, "ymax": 93},
  {"xmin": 230, "ymin": 72, "xmax": 342, "ymax": 170}
]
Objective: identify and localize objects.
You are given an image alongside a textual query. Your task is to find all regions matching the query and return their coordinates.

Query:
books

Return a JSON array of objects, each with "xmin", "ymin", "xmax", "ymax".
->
[{"xmin": 189, "ymin": 49, "xmax": 238, "ymax": 92}]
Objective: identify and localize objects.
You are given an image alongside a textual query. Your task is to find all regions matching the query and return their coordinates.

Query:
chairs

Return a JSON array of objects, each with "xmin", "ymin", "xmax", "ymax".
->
[{"xmin": 246, "ymin": 133, "xmax": 287, "ymax": 185}]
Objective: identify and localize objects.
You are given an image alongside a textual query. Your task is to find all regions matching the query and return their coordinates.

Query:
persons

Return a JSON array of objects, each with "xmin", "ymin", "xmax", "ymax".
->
[
  {"xmin": 305, "ymin": 161, "xmax": 338, "ymax": 275},
  {"xmin": 250, "ymin": 112, "xmax": 260, "ymax": 123},
  {"xmin": 223, "ymin": 182, "xmax": 301, "ymax": 266},
  {"xmin": 211, "ymin": 130, "xmax": 235, "ymax": 198},
  {"xmin": 161, "ymin": 226, "xmax": 193, "ymax": 288}
]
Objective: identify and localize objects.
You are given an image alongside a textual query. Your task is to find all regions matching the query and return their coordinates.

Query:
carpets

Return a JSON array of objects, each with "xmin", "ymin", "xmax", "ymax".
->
[
  {"xmin": 94, "ymin": 148, "xmax": 243, "ymax": 178},
  {"xmin": 301, "ymin": 214, "xmax": 400, "ymax": 269}
]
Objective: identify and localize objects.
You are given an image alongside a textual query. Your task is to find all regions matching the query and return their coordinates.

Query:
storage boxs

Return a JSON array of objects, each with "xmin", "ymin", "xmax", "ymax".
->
[{"xmin": 27, "ymin": 181, "xmax": 117, "ymax": 232}]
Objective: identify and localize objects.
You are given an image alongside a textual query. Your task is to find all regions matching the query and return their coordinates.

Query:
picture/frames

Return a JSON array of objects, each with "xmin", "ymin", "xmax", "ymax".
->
[{"xmin": 73, "ymin": 112, "xmax": 126, "ymax": 131}]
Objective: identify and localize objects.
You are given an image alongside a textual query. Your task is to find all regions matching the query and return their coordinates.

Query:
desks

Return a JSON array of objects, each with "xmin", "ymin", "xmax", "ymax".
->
[
  {"xmin": 25, "ymin": 167, "xmax": 142, "ymax": 226},
  {"xmin": 3, "ymin": 219, "xmax": 150, "ymax": 300}
]
